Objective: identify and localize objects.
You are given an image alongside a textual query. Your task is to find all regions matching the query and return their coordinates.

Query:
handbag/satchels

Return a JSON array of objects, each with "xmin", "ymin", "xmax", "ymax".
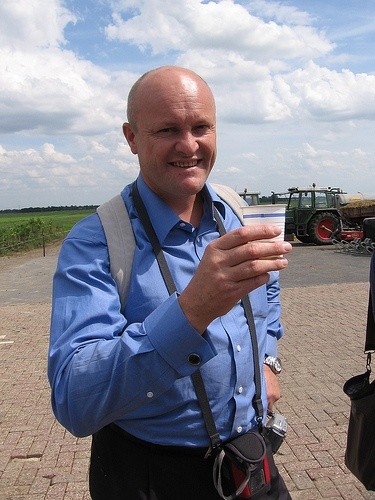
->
[
  {"xmin": 213, "ymin": 428, "xmax": 273, "ymax": 500},
  {"xmin": 343, "ymin": 371, "xmax": 375, "ymax": 492}
]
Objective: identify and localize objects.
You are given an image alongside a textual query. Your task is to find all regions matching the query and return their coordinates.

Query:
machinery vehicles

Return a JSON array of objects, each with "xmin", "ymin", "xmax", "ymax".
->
[{"xmin": 233, "ymin": 187, "xmax": 375, "ymax": 246}]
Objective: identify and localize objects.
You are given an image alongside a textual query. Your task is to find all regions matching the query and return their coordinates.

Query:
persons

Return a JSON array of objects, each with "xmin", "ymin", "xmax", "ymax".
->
[{"xmin": 47, "ymin": 65, "xmax": 293, "ymax": 500}]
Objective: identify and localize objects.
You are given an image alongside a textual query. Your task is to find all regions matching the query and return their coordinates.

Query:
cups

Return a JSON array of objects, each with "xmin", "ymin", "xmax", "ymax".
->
[{"xmin": 240, "ymin": 204, "xmax": 289, "ymax": 262}]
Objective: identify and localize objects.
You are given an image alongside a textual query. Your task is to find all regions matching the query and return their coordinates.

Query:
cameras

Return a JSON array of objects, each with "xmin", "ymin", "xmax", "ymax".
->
[{"xmin": 266, "ymin": 414, "xmax": 288, "ymax": 455}]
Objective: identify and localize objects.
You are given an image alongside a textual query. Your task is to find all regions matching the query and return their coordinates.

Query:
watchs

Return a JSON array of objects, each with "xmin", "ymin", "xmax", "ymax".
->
[{"xmin": 263, "ymin": 356, "xmax": 282, "ymax": 374}]
